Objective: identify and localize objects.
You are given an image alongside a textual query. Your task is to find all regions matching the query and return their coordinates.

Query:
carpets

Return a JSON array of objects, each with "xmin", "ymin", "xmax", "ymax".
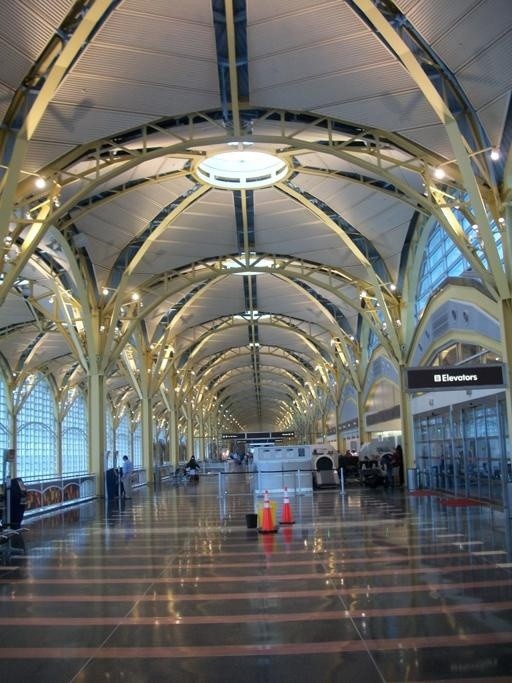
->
[{"xmin": 402, "ymin": 489, "xmax": 478, "ymax": 505}]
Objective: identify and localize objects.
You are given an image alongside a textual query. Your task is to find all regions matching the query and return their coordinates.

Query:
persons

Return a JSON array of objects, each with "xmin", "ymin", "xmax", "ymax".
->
[
  {"xmin": 184, "ymin": 455, "xmax": 201, "ymax": 470},
  {"xmin": 230, "ymin": 448, "xmax": 253, "ymax": 465},
  {"xmin": 121, "ymin": 455, "xmax": 133, "ymax": 499},
  {"xmin": 391, "ymin": 444, "xmax": 404, "ymax": 486},
  {"xmin": 337, "ymin": 448, "xmax": 358, "ymax": 467}
]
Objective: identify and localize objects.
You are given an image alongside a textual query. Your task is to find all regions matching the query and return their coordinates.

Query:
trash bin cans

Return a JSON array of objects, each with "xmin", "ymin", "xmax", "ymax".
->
[{"xmin": 407, "ymin": 468, "xmax": 419, "ymax": 490}]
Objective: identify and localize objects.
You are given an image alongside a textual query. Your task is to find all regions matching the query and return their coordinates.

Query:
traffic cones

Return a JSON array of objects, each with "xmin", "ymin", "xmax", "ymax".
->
[
  {"xmin": 257, "ymin": 492, "xmax": 279, "ymax": 531},
  {"xmin": 278, "ymin": 485, "xmax": 295, "ymax": 523}
]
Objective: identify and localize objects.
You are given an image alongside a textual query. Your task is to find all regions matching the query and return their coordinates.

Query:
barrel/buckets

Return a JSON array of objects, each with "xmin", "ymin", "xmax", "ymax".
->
[{"xmin": 245, "ymin": 513, "xmax": 257, "ymax": 528}]
